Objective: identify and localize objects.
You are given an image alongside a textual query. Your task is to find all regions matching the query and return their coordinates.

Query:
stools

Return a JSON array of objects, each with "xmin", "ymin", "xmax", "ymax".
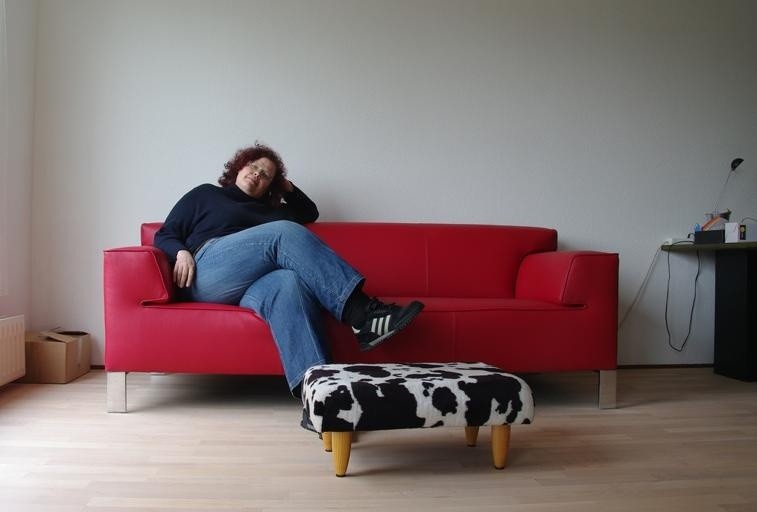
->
[{"xmin": 300, "ymin": 360, "xmax": 535, "ymax": 478}]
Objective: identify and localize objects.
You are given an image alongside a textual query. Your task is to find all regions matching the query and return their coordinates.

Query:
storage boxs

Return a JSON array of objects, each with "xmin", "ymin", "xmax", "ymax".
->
[{"xmin": 24, "ymin": 328, "xmax": 89, "ymax": 385}]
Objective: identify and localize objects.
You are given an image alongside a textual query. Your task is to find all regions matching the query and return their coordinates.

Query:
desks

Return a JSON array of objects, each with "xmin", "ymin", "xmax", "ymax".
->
[{"xmin": 661, "ymin": 234, "xmax": 754, "ymax": 381}]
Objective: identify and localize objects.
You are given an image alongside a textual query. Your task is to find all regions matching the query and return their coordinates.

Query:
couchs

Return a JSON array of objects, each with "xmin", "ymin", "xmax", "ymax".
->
[{"xmin": 98, "ymin": 222, "xmax": 620, "ymax": 412}]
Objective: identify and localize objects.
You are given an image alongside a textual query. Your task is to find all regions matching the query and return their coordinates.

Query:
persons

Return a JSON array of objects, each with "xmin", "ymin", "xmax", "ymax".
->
[{"xmin": 154, "ymin": 140, "xmax": 425, "ymax": 433}]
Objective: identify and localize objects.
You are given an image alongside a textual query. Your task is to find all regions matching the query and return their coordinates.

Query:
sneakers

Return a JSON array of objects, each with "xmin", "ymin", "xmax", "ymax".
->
[
  {"xmin": 352, "ymin": 299, "xmax": 425, "ymax": 353},
  {"xmin": 298, "ymin": 409, "xmax": 358, "ymax": 444}
]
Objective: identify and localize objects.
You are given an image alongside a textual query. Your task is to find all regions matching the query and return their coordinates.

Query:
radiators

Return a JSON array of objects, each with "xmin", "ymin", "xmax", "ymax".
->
[{"xmin": 0, "ymin": 314, "xmax": 28, "ymax": 385}]
